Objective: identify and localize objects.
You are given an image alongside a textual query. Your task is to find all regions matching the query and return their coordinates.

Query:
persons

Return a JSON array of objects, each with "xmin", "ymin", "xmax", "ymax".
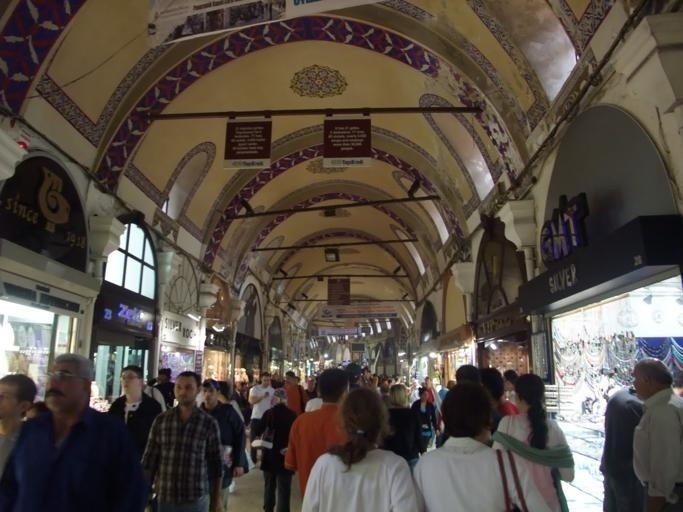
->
[
  {"xmin": 142, "ymin": 373, "xmax": 224, "ymax": 511},
  {"xmin": 457, "ymin": 367, "xmax": 501, "ymax": 440},
  {"xmin": 480, "ymin": 368, "xmax": 519, "ymax": 417},
  {"xmin": 436, "ymin": 379, "xmax": 455, "ymax": 448},
  {"xmin": 600, "ymin": 384, "xmax": 646, "ymax": 512},
  {"xmin": 493, "ymin": 374, "xmax": 544, "ymax": 450},
  {"xmin": 250, "ymin": 373, "xmax": 275, "ymax": 466},
  {"xmin": 344, "ymin": 362, "xmax": 392, "ymax": 408},
  {"xmin": 409, "ymin": 374, "xmax": 424, "ymax": 407},
  {"xmin": 284, "ymin": 368, "xmax": 350, "ymax": 505},
  {"xmin": 0, "ymin": 353, "xmax": 146, "ymax": 512},
  {"xmin": 257, "ymin": 389, "xmax": 299, "ymax": 512},
  {"xmin": 0, "ymin": 375, "xmax": 37, "ymax": 480},
  {"xmin": 631, "ymin": 357, "xmax": 683, "ymax": 512},
  {"xmin": 502, "ymin": 370, "xmax": 517, "ymax": 403},
  {"xmin": 411, "ymin": 389, "xmax": 440, "ymax": 456},
  {"xmin": 300, "ymin": 387, "xmax": 425, "ymax": 512},
  {"xmin": 25, "ymin": 401, "xmax": 47, "ymax": 422},
  {"xmin": 414, "ymin": 382, "xmax": 544, "ymax": 512},
  {"xmin": 274, "ymin": 369, "xmax": 323, "ymax": 413},
  {"xmin": 236, "ymin": 376, "xmax": 257, "ymax": 424},
  {"xmin": 144, "ymin": 368, "xmax": 179, "ymax": 410},
  {"xmin": 426, "ymin": 377, "xmax": 443, "ymax": 448},
  {"xmin": 199, "ymin": 377, "xmax": 246, "ymax": 512},
  {"xmin": 106, "ymin": 365, "xmax": 163, "ymax": 458},
  {"xmin": 192, "ymin": 379, "xmax": 245, "ymax": 420},
  {"xmin": 381, "ymin": 385, "xmax": 421, "ymax": 461}
]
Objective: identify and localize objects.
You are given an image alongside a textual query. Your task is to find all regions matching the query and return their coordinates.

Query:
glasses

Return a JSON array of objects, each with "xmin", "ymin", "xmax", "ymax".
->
[
  {"xmin": 121, "ymin": 375, "xmax": 142, "ymax": 381},
  {"xmin": 46, "ymin": 371, "xmax": 91, "ymax": 381}
]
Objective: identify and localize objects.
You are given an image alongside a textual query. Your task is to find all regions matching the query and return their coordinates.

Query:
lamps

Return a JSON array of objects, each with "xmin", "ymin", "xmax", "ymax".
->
[{"xmin": 325, "ymin": 249, "xmax": 339, "ymax": 262}]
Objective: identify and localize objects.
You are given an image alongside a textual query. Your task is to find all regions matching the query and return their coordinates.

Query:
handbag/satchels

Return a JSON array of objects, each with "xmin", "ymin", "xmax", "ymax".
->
[{"xmin": 251, "ymin": 410, "xmax": 277, "ymax": 449}]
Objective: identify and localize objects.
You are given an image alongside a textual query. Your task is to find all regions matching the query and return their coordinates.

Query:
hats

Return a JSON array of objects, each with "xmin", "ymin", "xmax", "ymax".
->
[{"xmin": 201, "ymin": 379, "xmax": 220, "ymax": 390}]
